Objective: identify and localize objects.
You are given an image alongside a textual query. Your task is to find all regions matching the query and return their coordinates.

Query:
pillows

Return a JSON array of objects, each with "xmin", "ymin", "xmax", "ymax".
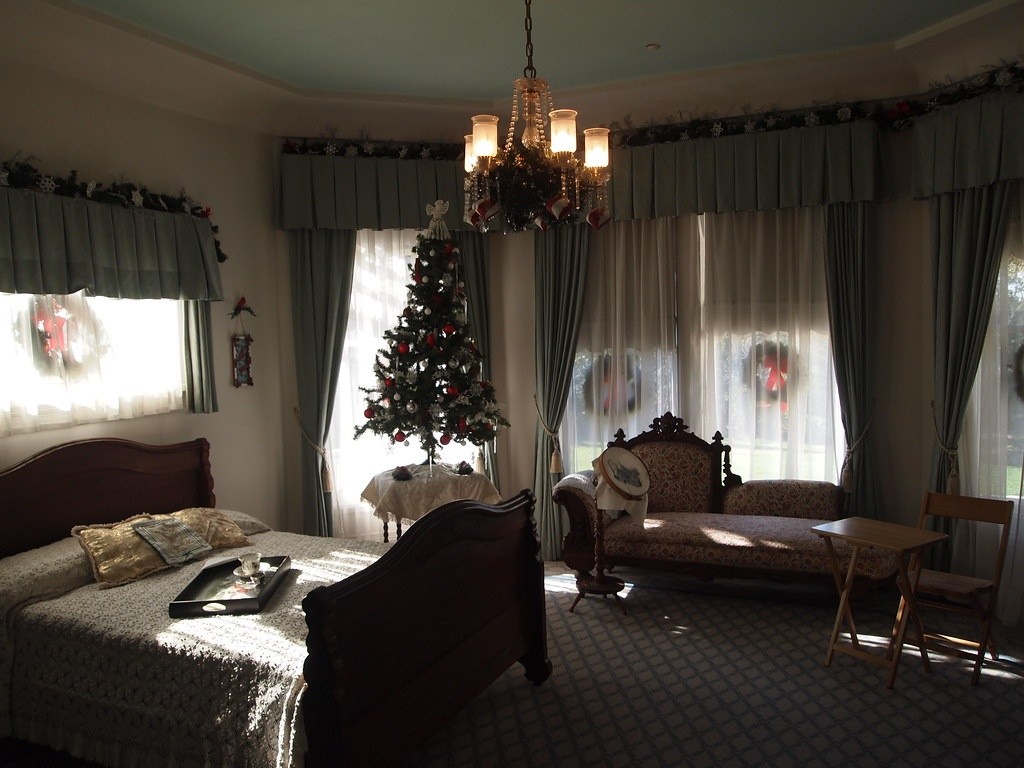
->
[
  {"xmin": 131, "ymin": 517, "xmax": 213, "ymax": 566},
  {"xmin": 71, "ymin": 512, "xmax": 185, "ymax": 590},
  {"xmin": 214, "ymin": 509, "xmax": 271, "ymax": 535},
  {"xmin": 170, "ymin": 506, "xmax": 248, "ymax": 549},
  {"xmin": 0, "ymin": 535, "xmax": 94, "ymax": 609},
  {"xmin": 723, "ymin": 479, "xmax": 844, "ymax": 522}
]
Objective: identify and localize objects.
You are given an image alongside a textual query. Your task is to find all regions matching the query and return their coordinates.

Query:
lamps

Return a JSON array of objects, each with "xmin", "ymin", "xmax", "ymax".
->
[{"xmin": 464, "ymin": 1, "xmax": 612, "ymax": 234}]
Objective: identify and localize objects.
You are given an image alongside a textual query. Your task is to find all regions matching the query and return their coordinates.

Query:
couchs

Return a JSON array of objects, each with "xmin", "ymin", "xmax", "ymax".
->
[{"xmin": 551, "ymin": 410, "xmax": 906, "ymax": 617}]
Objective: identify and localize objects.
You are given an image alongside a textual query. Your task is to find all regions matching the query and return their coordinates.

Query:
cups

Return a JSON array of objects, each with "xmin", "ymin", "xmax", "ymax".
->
[
  {"xmin": 238, "ymin": 553, "xmax": 261, "ymax": 574},
  {"xmin": 250, "ymin": 572, "xmax": 266, "ymax": 587}
]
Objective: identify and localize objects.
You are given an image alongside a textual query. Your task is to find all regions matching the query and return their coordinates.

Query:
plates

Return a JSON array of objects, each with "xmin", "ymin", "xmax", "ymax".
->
[{"xmin": 233, "ymin": 562, "xmax": 270, "ymax": 579}]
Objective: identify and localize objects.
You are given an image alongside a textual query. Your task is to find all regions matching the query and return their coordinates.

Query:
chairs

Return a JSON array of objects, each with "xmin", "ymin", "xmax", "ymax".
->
[{"xmin": 887, "ymin": 491, "xmax": 1014, "ymax": 685}]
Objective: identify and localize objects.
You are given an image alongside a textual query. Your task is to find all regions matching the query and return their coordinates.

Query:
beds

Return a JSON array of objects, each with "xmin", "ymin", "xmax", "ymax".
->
[{"xmin": 0, "ymin": 437, "xmax": 554, "ymax": 768}]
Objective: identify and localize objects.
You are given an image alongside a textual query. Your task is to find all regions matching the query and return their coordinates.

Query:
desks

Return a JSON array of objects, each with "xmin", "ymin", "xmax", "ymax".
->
[
  {"xmin": 812, "ymin": 516, "xmax": 949, "ymax": 687},
  {"xmin": 360, "ymin": 466, "xmax": 501, "ymax": 542}
]
